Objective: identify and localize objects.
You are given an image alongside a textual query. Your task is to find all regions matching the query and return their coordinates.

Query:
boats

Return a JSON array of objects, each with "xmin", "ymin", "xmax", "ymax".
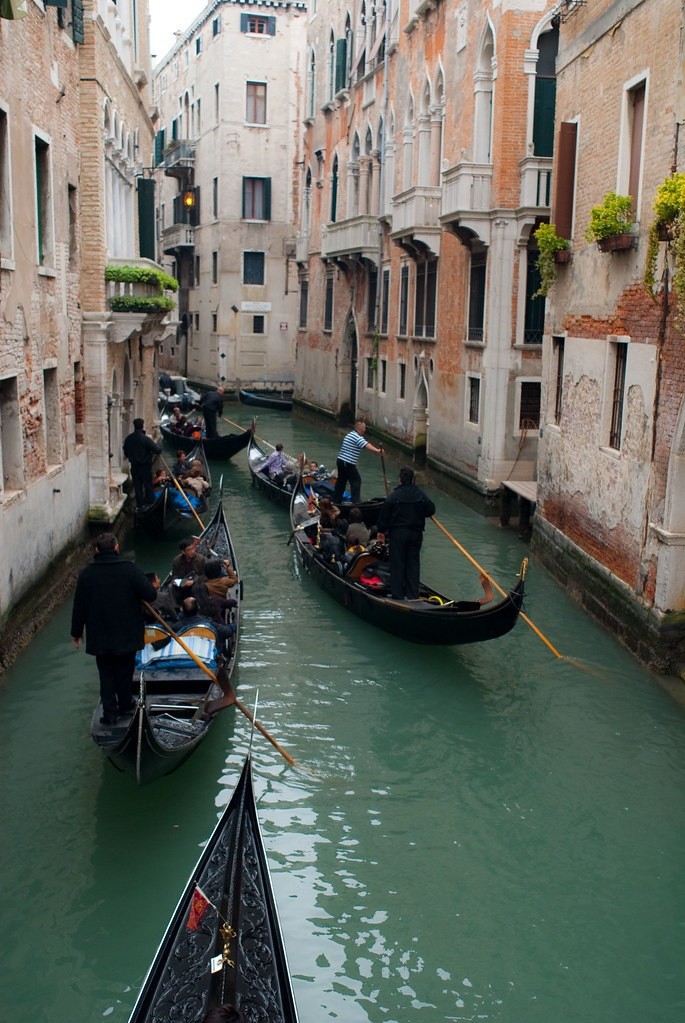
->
[
  {"xmin": 126, "ymin": 685, "xmax": 302, "ymax": 1023},
  {"xmin": 158, "ymin": 413, "xmax": 261, "ymax": 461},
  {"xmin": 131, "ymin": 435, "xmax": 212, "ymax": 544},
  {"xmin": 157, "ymin": 375, "xmax": 202, "ymax": 413},
  {"xmin": 238, "ymin": 387, "xmax": 295, "ymax": 413},
  {"xmin": 246, "ymin": 417, "xmax": 342, "ymax": 508},
  {"xmin": 290, "ymin": 449, "xmax": 528, "ymax": 646},
  {"xmin": 89, "ymin": 476, "xmax": 242, "ymax": 786}
]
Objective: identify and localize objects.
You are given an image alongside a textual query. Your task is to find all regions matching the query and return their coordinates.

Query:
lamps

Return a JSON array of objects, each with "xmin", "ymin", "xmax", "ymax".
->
[{"xmin": 138, "ymin": 165, "xmax": 196, "ymax": 213}]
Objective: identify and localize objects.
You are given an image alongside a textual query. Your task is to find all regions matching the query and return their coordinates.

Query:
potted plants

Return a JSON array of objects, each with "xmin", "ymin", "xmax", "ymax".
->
[{"xmin": 527, "ymin": 168, "xmax": 685, "ymax": 316}]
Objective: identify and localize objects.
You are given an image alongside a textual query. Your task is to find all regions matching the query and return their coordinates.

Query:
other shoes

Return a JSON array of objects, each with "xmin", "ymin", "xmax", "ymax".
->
[
  {"xmin": 100, "ymin": 715, "xmax": 115, "ymax": 725},
  {"xmin": 121, "ymin": 699, "xmax": 137, "ymax": 711}
]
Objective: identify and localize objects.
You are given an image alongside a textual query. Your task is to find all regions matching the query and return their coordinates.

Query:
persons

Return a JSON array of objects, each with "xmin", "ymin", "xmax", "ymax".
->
[
  {"xmin": 169, "ymin": 407, "xmax": 206, "ymax": 437},
  {"xmin": 160, "ymin": 371, "xmax": 176, "ymax": 395},
  {"xmin": 71, "ymin": 532, "xmax": 157, "ymax": 726},
  {"xmin": 377, "ymin": 467, "xmax": 436, "ymax": 600},
  {"xmin": 314, "ymin": 498, "xmax": 388, "ymax": 575},
  {"xmin": 123, "ymin": 418, "xmax": 161, "ymax": 510},
  {"xmin": 141, "ymin": 537, "xmax": 237, "ymax": 660},
  {"xmin": 152, "ymin": 450, "xmax": 209, "ymax": 498},
  {"xmin": 195, "ymin": 387, "xmax": 225, "ymax": 438},
  {"xmin": 255, "ymin": 444, "xmax": 330, "ymax": 494},
  {"xmin": 332, "ymin": 422, "xmax": 385, "ymax": 504}
]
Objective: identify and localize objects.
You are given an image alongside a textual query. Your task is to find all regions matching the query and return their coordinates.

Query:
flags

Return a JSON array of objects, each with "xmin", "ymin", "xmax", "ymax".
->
[{"xmin": 186, "ymin": 886, "xmax": 217, "ymax": 930}]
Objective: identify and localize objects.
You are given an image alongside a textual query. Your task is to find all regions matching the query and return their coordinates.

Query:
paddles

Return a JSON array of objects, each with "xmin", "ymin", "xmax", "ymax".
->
[
  {"xmin": 158, "ymin": 454, "xmax": 206, "ymax": 532},
  {"xmin": 194, "ymin": 401, "xmax": 298, "ymax": 463},
  {"xmin": 143, "ymin": 597, "xmax": 296, "ymax": 766},
  {"xmin": 431, "ymin": 514, "xmax": 594, "ymax": 674},
  {"xmin": 377, "ymin": 443, "xmax": 390, "ymax": 494}
]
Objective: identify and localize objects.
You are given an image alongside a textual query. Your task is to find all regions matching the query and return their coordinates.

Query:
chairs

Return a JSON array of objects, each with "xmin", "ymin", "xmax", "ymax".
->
[
  {"xmin": 344, "ymin": 551, "xmax": 381, "ymax": 580},
  {"xmin": 144, "ymin": 621, "xmax": 218, "ymax": 643}
]
